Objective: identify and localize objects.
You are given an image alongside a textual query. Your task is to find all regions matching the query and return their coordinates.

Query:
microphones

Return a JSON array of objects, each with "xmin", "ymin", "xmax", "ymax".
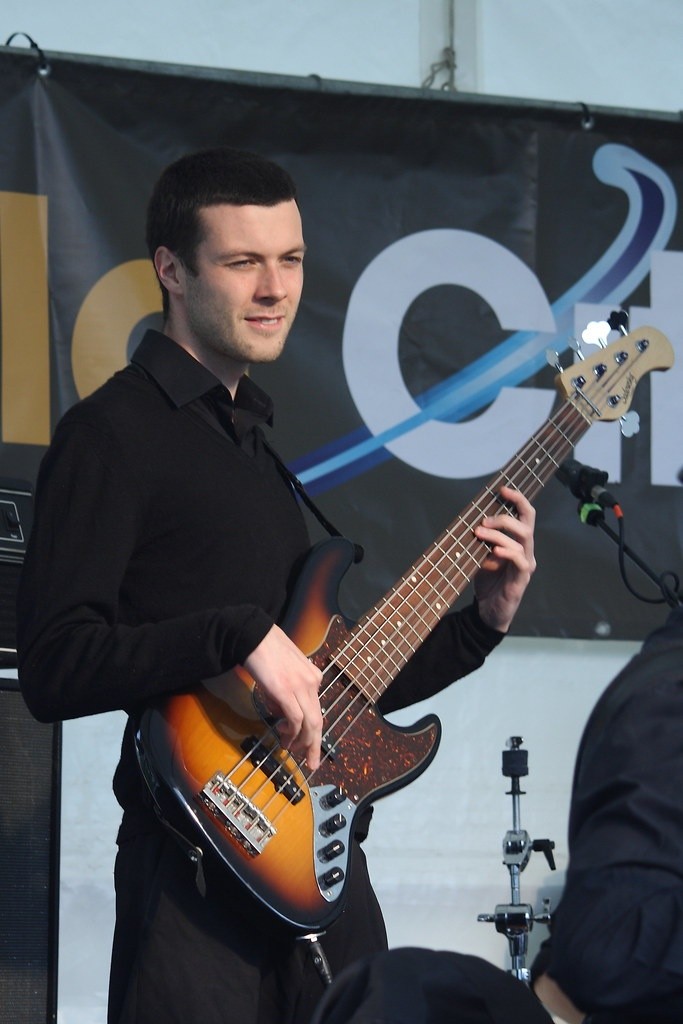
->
[{"xmin": 555, "ymin": 460, "xmax": 619, "ymax": 509}]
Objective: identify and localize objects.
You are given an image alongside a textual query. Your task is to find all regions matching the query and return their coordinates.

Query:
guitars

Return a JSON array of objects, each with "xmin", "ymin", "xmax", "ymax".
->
[{"xmin": 130, "ymin": 302, "xmax": 676, "ymax": 943}]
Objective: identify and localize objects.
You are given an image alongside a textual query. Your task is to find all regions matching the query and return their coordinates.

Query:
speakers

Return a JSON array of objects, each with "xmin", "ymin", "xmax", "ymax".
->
[{"xmin": 0, "ymin": 680, "xmax": 58, "ymax": 1024}]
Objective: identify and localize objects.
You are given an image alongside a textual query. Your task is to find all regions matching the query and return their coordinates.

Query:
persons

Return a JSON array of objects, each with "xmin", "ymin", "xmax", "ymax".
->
[
  {"xmin": 532, "ymin": 609, "xmax": 683, "ymax": 1024},
  {"xmin": 17, "ymin": 150, "xmax": 536, "ymax": 1024}
]
